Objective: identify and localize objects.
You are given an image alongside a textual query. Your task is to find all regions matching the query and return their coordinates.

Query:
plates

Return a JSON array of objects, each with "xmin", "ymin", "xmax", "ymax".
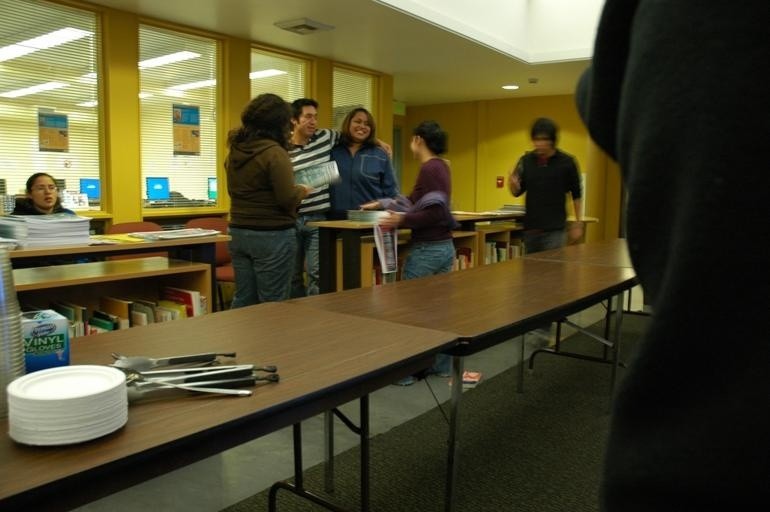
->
[{"xmin": 5, "ymin": 364, "xmax": 128, "ymax": 447}]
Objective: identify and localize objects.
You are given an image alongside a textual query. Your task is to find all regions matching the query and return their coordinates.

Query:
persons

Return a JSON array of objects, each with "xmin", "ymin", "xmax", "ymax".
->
[
  {"xmin": 331, "ymin": 108, "xmax": 399, "ymax": 216},
  {"xmin": 358, "ymin": 120, "xmax": 461, "ymax": 387},
  {"xmin": 9, "ymin": 173, "xmax": 75, "ymax": 215},
  {"xmin": 573, "ymin": 0, "xmax": 770, "ymax": 512},
  {"xmin": 228, "ymin": 94, "xmax": 317, "ymax": 309},
  {"xmin": 290, "ymin": 98, "xmax": 393, "ymax": 297},
  {"xmin": 506, "ymin": 117, "xmax": 586, "ymax": 253}
]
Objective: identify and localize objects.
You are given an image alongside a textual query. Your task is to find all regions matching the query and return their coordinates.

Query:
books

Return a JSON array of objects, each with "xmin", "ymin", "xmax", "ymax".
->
[
  {"xmin": 448, "ymin": 370, "xmax": 483, "ymax": 389},
  {"xmin": 349, "ymin": 205, "xmax": 525, "ymax": 284},
  {"xmin": 1, "ymin": 212, "xmax": 221, "ymax": 337}
]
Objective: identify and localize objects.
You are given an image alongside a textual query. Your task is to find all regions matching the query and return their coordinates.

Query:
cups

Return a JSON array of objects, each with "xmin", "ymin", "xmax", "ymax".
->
[{"xmin": 1, "ymin": 249, "xmax": 26, "ymax": 422}]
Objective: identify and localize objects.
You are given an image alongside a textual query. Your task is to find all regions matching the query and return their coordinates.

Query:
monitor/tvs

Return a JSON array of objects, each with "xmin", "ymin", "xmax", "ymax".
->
[
  {"xmin": 48, "ymin": 176, "xmax": 68, "ymax": 196},
  {"xmin": 206, "ymin": 175, "xmax": 221, "ymax": 202},
  {"xmin": 0, "ymin": 175, "xmax": 10, "ymax": 199},
  {"xmin": 80, "ymin": 177, "xmax": 101, "ymax": 202},
  {"xmin": 145, "ymin": 175, "xmax": 171, "ymax": 204}
]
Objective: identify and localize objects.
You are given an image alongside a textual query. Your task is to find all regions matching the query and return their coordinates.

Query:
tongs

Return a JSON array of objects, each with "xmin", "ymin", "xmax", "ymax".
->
[{"xmin": 111, "ymin": 351, "xmax": 280, "ymax": 403}]
